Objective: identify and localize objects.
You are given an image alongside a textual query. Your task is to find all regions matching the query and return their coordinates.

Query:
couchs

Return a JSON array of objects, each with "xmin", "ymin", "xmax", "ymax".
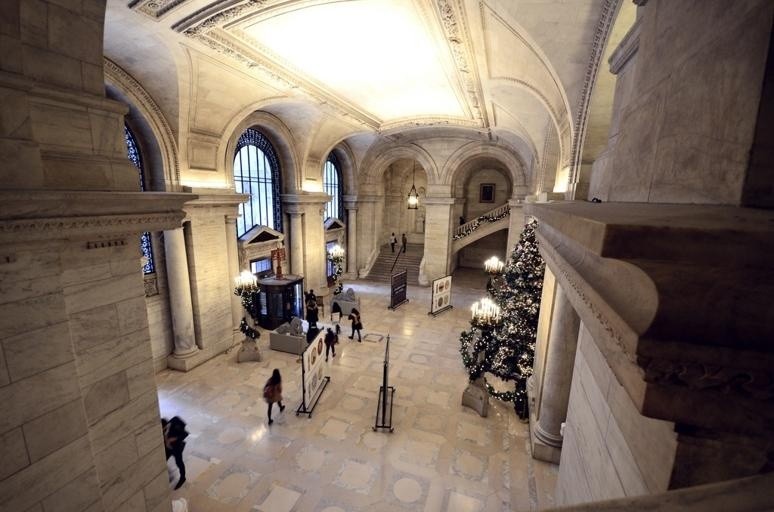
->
[
  {"xmin": 268, "ymin": 317, "xmax": 310, "ymax": 355},
  {"xmin": 328, "ymin": 288, "xmax": 361, "ymax": 316}
]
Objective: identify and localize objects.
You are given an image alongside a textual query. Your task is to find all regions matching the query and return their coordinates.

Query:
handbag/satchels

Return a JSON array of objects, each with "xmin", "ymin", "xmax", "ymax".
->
[{"xmin": 263, "ymin": 386, "xmax": 272, "ymax": 398}]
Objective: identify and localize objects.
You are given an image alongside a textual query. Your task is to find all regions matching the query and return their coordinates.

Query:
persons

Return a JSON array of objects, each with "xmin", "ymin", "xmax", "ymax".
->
[
  {"xmin": 262, "ymin": 369, "xmax": 285, "ymax": 425},
  {"xmin": 324, "ymin": 328, "xmax": 336, "ymax": 363},
  {"xmin": 331, "ymin": 302, "xmax": 343, "ymax": 335},
  {"xmin": 348, "ymin": 308, "xmax": 362, "ymax": 342},
  {"xmin": 459, "ymin": 216, "xmax": 465, "ymax": 225},
  {"xmin": 304, "ymin": 289, "xmax": 320, "ymax": 344},
  {"xmin": 402, "ymin": 234, "xmax": 407, "ymax": 253},
  {"xmin": 162, "ymin": 416, "xmax": 188, "ymax": 490},
  {"xmin": 390, "ymin": 232, "xmax": 396, "ymax": 253}
]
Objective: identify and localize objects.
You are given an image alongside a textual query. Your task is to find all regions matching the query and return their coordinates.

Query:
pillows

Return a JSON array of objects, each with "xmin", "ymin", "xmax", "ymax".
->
[
  {"xmin": 274, "ymin": 318, "xmax": 305, "ymax": 338},
  {"xmin": 335, "ymin": 288, "xmax": 356, "ymax": 303}
]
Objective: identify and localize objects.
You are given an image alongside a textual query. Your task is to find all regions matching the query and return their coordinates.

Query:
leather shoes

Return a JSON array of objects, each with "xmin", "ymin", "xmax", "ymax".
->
[{"xmin": 174, "ymin": 478, "xmax": 186, "ymax": 490}]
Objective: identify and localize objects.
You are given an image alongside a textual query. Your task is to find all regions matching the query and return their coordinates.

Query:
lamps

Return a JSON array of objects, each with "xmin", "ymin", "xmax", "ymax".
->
[{"xmin": 404, "ymin": 159, "xmax": 419, "ymax": 210}]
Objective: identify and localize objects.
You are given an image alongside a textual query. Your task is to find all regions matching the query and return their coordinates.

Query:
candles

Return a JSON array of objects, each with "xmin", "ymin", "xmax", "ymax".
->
[
  {"xmin": 233, "ymin": 270, "xmax": 259, "ymax": 290},
  {"xmin": 329, "ymin": 245, "xmax": 346, "ymax": 261},
  {"xmin": 483, "ymin": 256, "xmax": 505, "ymax": 276},
  {"xmin": 469, "ymin": 296, "xmax": 503, "ymax": 327}
]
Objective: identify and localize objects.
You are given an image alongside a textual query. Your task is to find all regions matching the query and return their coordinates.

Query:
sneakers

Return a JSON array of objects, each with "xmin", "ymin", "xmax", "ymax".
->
[
  {"xmin": 280, "ymin": 405, "xmax": 285, "ymax": 411},
  {"xmin": 268, "ymin": 419, "xmax": 273, "ymax": 424}
]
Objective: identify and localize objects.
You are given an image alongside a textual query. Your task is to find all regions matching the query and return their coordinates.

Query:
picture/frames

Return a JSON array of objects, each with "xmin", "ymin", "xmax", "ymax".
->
[{"xmin": 478, "ymin": 183, "xmax": 496, "ymax": 203}]
